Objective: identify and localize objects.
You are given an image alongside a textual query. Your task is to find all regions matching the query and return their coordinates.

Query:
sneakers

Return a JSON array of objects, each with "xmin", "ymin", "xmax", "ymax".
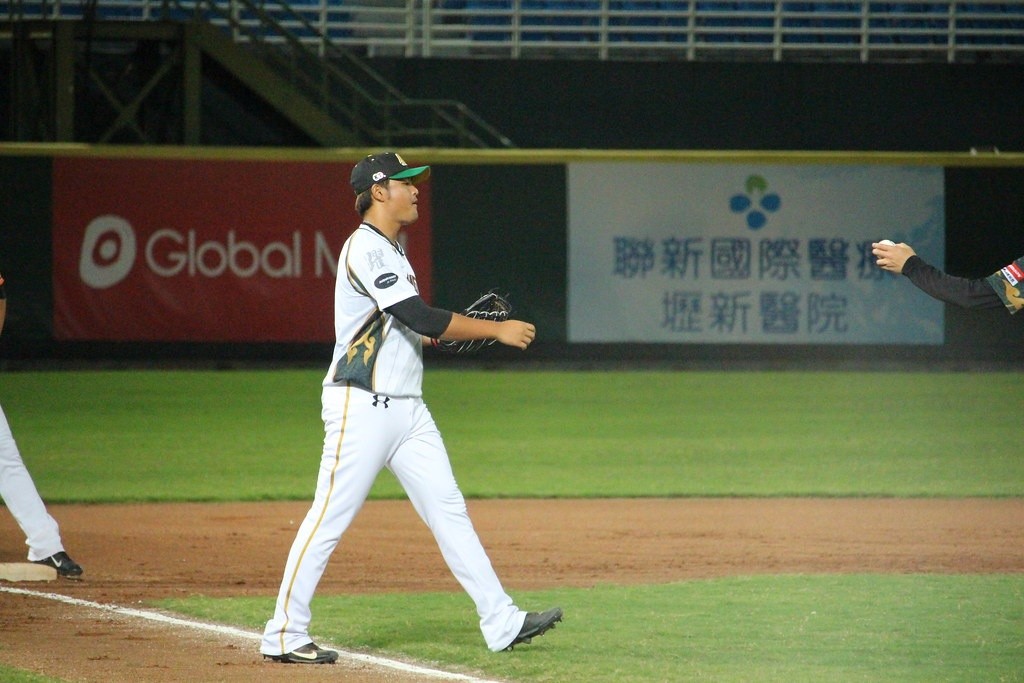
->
[
  {"xmin": 504, "ymin": 607, "xmax": 563, "ymax": 651},
  {"xmin": 264, "ymin": 643, "xmax": 338, "ymax": 664},
  {"xmin": 36, "ymin": 551, "xmax": 83, "ymax": 576}
]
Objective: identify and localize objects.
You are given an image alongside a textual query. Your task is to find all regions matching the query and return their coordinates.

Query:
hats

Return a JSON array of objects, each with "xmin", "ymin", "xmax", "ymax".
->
[{"xmin": 350, "ymin": 152, "xmax": 431, "ymax": 195}]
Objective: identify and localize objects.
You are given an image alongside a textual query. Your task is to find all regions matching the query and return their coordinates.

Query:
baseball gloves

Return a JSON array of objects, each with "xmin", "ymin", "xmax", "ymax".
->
[{"xmin": 437, "ymin": 293, "xmax": 512, "ymax": 354}]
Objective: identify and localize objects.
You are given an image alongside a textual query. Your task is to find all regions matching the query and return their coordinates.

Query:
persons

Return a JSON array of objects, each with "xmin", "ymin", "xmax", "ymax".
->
[
  {"xmin": 260, "ymin": 153, "xmax": 562, "ymax": 666},
  {"xmin": 872, "ymin": 240, "xmax": 1024, "ymax": 315},
  {"xmin": 0, "ymin": 274, "xmax": 85, "ymax": 577}
]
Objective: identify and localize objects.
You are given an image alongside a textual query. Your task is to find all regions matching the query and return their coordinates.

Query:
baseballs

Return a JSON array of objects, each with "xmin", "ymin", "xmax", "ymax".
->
[{"xmin": 877, "ymin": 238, "xmax": 896, "ymax": 259}]
